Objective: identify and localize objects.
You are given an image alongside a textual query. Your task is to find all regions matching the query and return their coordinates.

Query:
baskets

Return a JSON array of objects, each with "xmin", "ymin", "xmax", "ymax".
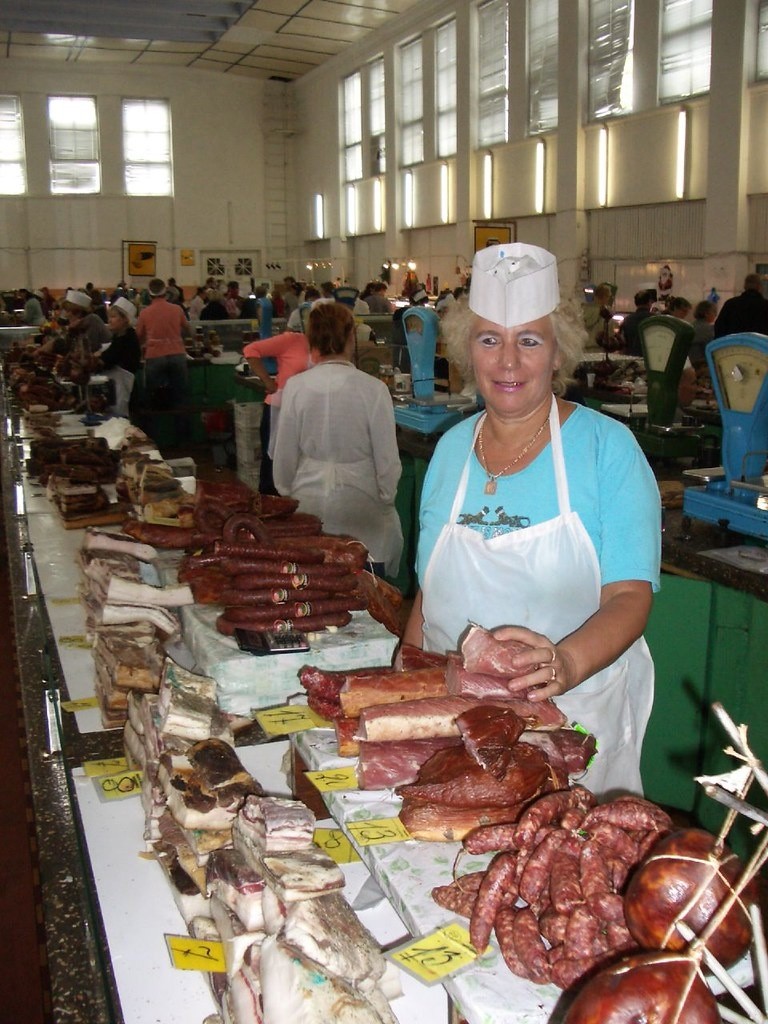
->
[{"xmin": 233, "ymin": 401, "xmax": 265, "ymax": 490}]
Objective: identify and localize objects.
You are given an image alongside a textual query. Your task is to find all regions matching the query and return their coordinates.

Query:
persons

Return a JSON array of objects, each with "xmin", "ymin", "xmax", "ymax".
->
[
  {"xmin": 395, "ymin": 242, "xmax": 663, "ymax": 802},
  {"xmin": 244, "ymin": 310, "xmax": 314, "ymax": 497},
  {"xmin": 714, "ymin": 274, "xmax": 768, "ymax": 339},
  {"xmin": 665, "ymin": 297, "xmax": 690, "ymax": 320},
  {"xmin": 17, "ymin": 277, "xmax": 197, "ymax": 452},
  {"xmin": 580, "ymin": 285, "xmax": 617, "ymax": 354},
  {"xmin": 189, "ymin": 278, "xmax": 287, "ymax": 321},
  {"xmin": 321, "ymin": 281, "xmax": 335, "ymax": 298},
  {"xmin": 273, "ymin": 304, "xmax": 404, "ymax": 585},
  {"xmin": 688, "ymin": 301, "xmax": 718, "ymax": 371},
  {"xmin": 410, "ymin": 284, "xmax": 429, "ymax": 307},
  {"xmin": 619, "ymin": 291, "xmax": 654, "ymax": 357},
  {"xmin": 435, "ymin": 279, "xmax": 471, "ymax": 311},
  {"xmin": 353, "ymin": 282, "xmax": 397, "ymax": 313},
  {"xmin": 284, "ymin": 277, "xmax": 304, "ymax": 321},
  {"xmin": 287, "ymin": 289, "xmax": 321, "ymax": 333}
]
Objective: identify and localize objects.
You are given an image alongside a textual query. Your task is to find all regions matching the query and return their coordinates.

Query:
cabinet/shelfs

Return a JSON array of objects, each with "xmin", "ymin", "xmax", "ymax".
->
[
  {"xmin": 190, "ymin": 361, "xmax": 267, "ymax": 441},
  {"xmin": 640, "ymin": 571, "xmax": 768, "ymax": 859},
  {"xmin": 390, "ymin": 455, "xmax": 428, "ymax": 602}
]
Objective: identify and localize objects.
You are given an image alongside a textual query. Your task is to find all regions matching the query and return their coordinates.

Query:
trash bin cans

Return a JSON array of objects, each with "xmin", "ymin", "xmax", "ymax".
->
[{"xmin": 631, "ymin": 314, "xmax": 724, "ymax": 470}]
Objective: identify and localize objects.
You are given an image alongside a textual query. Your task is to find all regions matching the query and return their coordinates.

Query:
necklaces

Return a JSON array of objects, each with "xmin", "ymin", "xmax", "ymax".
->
[{"xmin": 479, "ymin": 418, "xmax": 548, "ymax": 494}]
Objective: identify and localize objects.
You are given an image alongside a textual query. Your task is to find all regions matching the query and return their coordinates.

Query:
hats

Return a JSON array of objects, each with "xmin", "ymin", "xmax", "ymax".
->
[
  {"xmin": 113, "ymin": 297, "xmax": 137, "ymax": 320},
  {"xmin": 65, "ymin": 290, "xmax": 92, "ymax": 308},
  {"xmin": 34, "ymin": 290, "xmax": 44, "ymax": 299},
  {"xmin": 311, "ymin": 298, "xmax": 335, "ymax": 312},
  {"xmin": 468, "ymin": 242, "xmax": 560, "ymax": 328}
]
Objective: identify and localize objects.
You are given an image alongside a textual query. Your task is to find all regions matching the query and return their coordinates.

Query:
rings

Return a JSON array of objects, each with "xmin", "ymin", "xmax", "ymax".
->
[
  {"xmin": 545, "ymin": 666, "xmax": 556, "ymax": 684},
  {"xmin": 541, "ymin": 650, "xmax": 556, "ymax": 665}
]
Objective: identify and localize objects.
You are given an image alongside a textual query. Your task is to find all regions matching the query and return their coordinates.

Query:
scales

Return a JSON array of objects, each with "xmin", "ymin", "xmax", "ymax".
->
[
  {"xmin": 244, "ymin": 296, "xmax": 278, "ymax": 378},
  {"xmin": 681, "ymin": 331, "xmax": 768, "ymax": 542},
  {"xmin": 394, "ymin": 306, "xmax": 466, "ymax": 441},
  {"xmin": 299, "ymin": 304, "xmax": 312, "ymax": 334},
  {"xmin": 332, "ymin": 287, "xmax": 360, "ymax": 311}
]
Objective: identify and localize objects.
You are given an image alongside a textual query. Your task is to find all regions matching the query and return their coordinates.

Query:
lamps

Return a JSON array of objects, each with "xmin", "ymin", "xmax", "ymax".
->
[
  {"xmin": 441, "ymin": 161, "xmax": 449, "ymax": 225},
  {"xmin": 484, "ymin": 150, "xmax": 494, "ymax": 220},
  {"xmin": 406, "ymin": 168, "xmax": 413, "ymax": 228},
  {"xmin": 535, "ymin": 138, "xmax": 545, "ymax": 214},
  {"xmin": 676, "ymin": 104, "xmax": 686, "ymax": 201},
  {"xmin": 315, "ymin": 193, "xmax": 324, "ymax": 240},
  {"xmin": 374, "ymin": 177, "xmax": 382, "ymax": 232},
  {"xmin": 598, "ymin": 122, "xmax": 608, "ymax": 207},
  {"xmin": 349, "ymin": 183, "xmax": 356, "ymax": 237}
]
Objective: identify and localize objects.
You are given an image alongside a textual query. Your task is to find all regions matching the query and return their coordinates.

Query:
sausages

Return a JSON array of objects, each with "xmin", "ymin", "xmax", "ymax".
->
[
  {"xmin": 431, "ymin": 786, "xmax": 682, "ymax": 991},
  {"xmin": 177, "ymin": 500, "xmax": 363, "ymax": 637}
]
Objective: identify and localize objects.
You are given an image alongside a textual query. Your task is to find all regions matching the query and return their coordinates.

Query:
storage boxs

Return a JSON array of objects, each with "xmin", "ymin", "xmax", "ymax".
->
[{"xmin": 235, "ymin": 401, "xmax": 264, "ymax": 491}]
